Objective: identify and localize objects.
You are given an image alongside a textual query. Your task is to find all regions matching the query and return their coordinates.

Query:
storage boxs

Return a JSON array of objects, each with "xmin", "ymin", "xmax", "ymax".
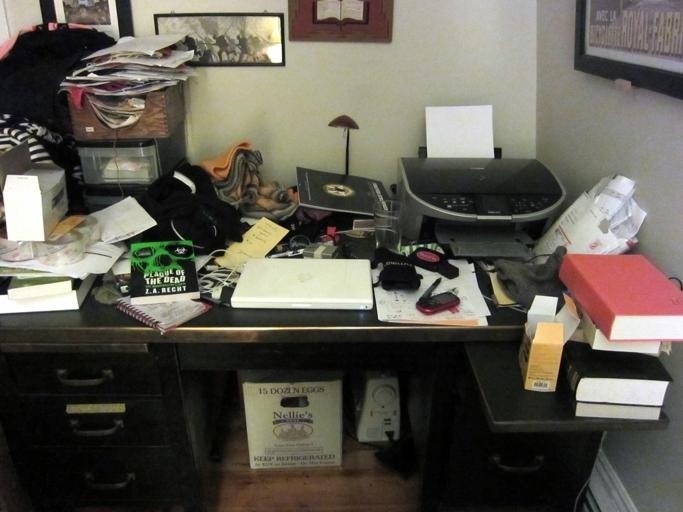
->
[
  {"xmin": 67, "ymin": 79, "xmax": 187, "ymax": 215},
  {"xmin": 347, "ymin": 376, "xmax": 402, "ymax": 443},
  {"xmin": 240, "ymin": 365, "xmax": 345, "ymax": 469}
]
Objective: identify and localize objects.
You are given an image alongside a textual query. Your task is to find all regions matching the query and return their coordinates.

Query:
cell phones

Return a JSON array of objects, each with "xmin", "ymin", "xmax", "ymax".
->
[{"xmin": 416, "ymin": 292, "xmax": 460, "ymax": 315}]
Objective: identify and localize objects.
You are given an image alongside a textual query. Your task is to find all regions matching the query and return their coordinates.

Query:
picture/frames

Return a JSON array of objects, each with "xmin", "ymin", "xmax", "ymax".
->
[
  {"xmin": 287, "ymin": 0, "xmax": 393, "ymax": 42},
  {"xmin": 154, "ymin": 13, "xmax": 285, "ymax": 66},
  {"xmin": 39, "ymin": 0, "xmax": 134, "ymax": 41},
  {"xmin": 573, "ymin": 0, "xmax": 683, "ymax": 101}
]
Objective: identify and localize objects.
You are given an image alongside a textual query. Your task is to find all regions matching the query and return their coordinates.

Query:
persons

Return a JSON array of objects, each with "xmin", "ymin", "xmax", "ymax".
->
[{"xmin": 185, "ymin": 32, "xmax": 271, "ymax": 65}]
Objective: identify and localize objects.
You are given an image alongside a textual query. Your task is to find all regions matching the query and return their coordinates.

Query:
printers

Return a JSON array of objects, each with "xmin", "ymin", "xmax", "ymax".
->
[{"xmin": 395, "ymin": 146, "xmax": 567, "ymax": 261}]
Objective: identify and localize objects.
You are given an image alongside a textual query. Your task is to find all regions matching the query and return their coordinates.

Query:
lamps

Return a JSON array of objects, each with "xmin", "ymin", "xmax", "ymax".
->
[{"xmin": 328, "ymin": 114, "xmax": 360, "ymax": 175}]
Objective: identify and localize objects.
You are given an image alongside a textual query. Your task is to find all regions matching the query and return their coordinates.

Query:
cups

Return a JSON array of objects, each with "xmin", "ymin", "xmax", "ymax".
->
[{"xmin": 373, "ymin": 200, "xmax": 404, "ymax": 253}]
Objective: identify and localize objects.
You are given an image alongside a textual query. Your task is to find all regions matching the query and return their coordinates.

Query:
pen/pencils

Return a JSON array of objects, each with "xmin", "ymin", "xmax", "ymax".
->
[{"xmin": 419, "ymin": 277, "xmax": 441, "ymax": 301}]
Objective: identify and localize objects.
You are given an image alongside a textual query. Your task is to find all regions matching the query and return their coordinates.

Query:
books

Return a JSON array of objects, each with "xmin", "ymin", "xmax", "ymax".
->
[
  {"xmin": 580, "ymin": 305, "xmax": 660, "ymax": 353},
  {"xmin": 556, "ymin": 377, "xmax": 661, "ymax": 419},
  {"xmin": 560, "ymin": 339, "xmax": 673, "ymax": 406},
  {"xmin": 128, "ymin": 239, "xmax": 199, "ymax": 305},
  {"xmin": 6, "ymin": 276, "xmax": 72, "ymax": 302},
  {"xmin": 558, "ymin": 254, "xmax": 682, "ymax": 341},
  {"xmin": 294, "ymin": 164, "xmax": 390, "ymax": 217},
  {"xmin": 0, "ymin": 272, "xmax": 97, "ymax": 315},
  {"xmin": 114, "ymin": 295, "xmax": 214, "ymax": 332}
]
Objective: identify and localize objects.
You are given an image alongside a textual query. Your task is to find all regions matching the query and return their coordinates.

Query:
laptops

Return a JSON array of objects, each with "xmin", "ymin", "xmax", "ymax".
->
[{"xmin": 230, "ymin": 258, "xmax": 374, "ymax": 311}]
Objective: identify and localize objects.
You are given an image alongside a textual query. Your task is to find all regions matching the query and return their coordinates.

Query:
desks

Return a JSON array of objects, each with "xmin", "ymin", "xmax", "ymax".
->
[{"xmin": 1, "ymin": 239, "xmax": 671, "ymax": 511}]
